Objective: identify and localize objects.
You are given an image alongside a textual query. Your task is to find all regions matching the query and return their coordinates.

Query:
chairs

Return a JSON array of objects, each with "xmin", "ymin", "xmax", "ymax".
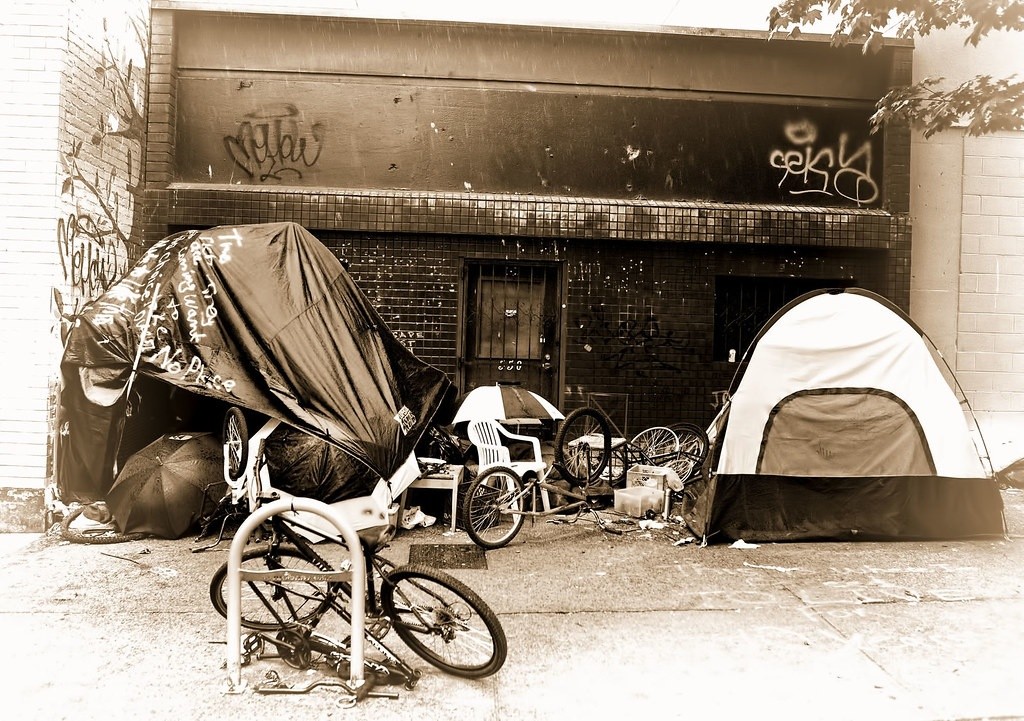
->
[
  {"xmin": 398, "ymin": 465, "xmax": 464, "ymax": 532},
  {"xmin": 468, "ymin": 419, "xmax": 551, "ymax": 524}
]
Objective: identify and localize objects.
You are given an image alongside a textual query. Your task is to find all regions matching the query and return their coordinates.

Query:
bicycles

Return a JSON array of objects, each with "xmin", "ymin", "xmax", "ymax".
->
[
  {"xmin": 463, "ymin": 407, "xmax": 625, "ymax": 548},
  {"xmin": 210, "ymin": 442, "xmax": 509, "ymax": 678}
]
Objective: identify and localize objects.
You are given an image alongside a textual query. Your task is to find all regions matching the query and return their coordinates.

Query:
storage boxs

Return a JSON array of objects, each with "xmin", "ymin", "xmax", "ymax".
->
[
  {"xmin": 626, "ymin": 464, "xmax": 672, "ymax": 489},
  {"xmin": 614, "ymin": 486, "xmax": 665, "ymax": 517}
]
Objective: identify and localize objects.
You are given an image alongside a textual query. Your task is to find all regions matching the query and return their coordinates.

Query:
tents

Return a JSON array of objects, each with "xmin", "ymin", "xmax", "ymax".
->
[{"xmin": 680, "ymin": 287, "xmax": 1010, "ymax": 550}]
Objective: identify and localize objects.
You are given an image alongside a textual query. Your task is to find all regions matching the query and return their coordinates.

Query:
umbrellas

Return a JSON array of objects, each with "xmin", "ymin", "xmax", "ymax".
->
[
  {"xmin": 440, "ymin": 382, "xmax": 566, "ymax": 421},
  {"xmin": 105, "ymin": 430, "xmax": 228, "ymax": 538},
  {"xmin": 248, "ymin": 416, "xmax": 422, "ymax": 543}
]
